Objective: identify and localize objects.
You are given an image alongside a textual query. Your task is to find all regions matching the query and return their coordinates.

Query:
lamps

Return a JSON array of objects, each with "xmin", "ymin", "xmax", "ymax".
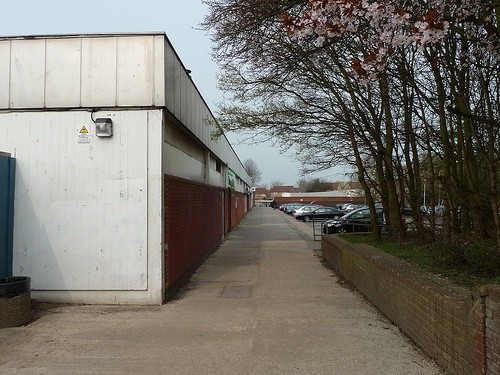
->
[{"xmin": 94, "ymin": 118, "xmax": 112, "ymax": 138}]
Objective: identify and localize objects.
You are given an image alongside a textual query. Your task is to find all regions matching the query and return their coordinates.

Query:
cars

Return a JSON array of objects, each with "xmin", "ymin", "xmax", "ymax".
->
[
  {"xmin": 277, "ymin": 202, "xmax": 452, "ymax": 219},
  {"xmin": 322, "ymin": 206, "xmax": 408, "ymax": 235},
  {"xmin": 295, "ymin": 207, "xmax": 346, "ymax": 222}
]
